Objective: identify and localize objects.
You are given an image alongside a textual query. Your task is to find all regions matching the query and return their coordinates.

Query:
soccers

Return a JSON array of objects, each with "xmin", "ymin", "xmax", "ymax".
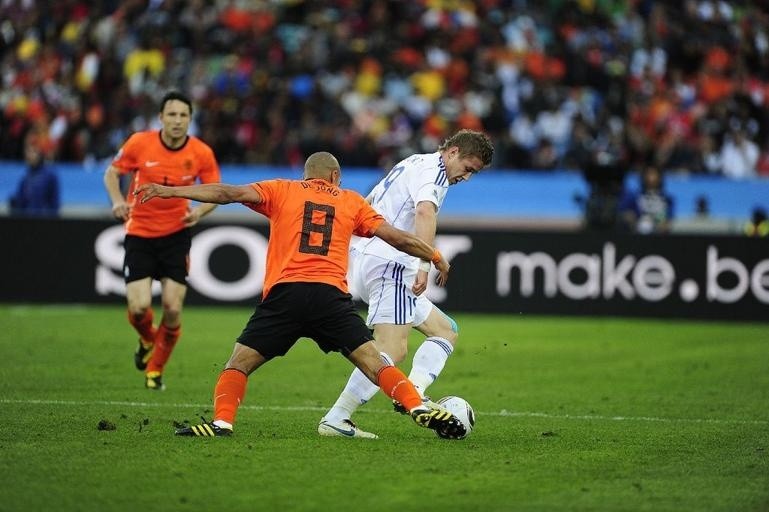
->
[{"xmin": 436, "ymin": 396, "xmax": 474, "ymax": 439}]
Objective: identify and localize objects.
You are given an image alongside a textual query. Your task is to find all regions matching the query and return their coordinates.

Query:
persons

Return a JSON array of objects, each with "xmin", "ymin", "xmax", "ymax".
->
[
  {"xmin": 317, "ymin": 128, "xmax": 493, "ymax": 440},
  {"xmin": 104, "ymin": 90, "xmax": 221, "ymax": 389},
  {"xmin": 1, "ymin": 0, "xmax": 769, "ymax": 237},
  {"xmin": 132, "ymin": 151, "xmax": 468, "ymax": 440}
]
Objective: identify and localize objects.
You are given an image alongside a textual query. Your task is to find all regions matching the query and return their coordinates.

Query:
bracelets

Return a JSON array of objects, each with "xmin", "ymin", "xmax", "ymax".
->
[{"xmin": 429, "ymin": 249, "xmax": 444, "ymax": 264}]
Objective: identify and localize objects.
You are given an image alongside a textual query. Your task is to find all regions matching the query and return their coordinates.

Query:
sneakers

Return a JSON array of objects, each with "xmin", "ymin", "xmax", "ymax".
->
[
  {"xmin": 393, "ymin": 393, "xmax": 468, "ymax": 438},
  {"xmin": 176, "ymin": 422, "xmax": 233, "ymax": 438},
  {"xmin": 145, "ymin": 373, "xmax": 164, "ymax": 388},
  {"xmin": 318, "ymin": 416, "xmax": 378, "ymax": 440},
  {"xmin": 134, "ymin": 325, "xmax": 157, "ymax": 371}
]
